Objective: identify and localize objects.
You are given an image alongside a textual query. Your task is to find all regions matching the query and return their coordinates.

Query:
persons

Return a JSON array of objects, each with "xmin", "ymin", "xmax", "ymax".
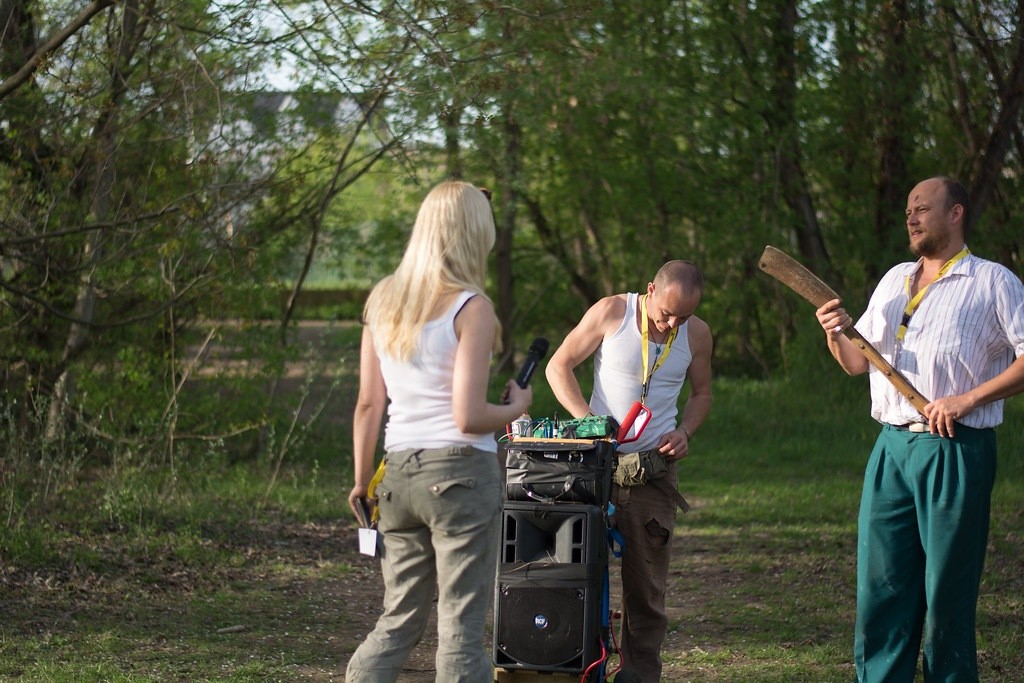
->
[
  {"xmin": 544, "ymin": 258, "xmax": 714, "ymax": 682},
  {"xmin": 345, "ymin": 182, "xmax": 534, "ymax": 683},
  {"xmin": 816, "ymin": 175, "xmax": 1023, "ymax": 683}
]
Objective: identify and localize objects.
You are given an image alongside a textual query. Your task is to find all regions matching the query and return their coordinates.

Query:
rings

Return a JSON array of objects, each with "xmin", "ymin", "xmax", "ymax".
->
[{"xmin": 836, "ymin": 326, "xmax": 842, "ymax": 333}]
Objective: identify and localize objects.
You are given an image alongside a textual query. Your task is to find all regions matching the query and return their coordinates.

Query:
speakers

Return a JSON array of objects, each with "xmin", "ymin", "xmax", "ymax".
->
[{"xmin": 494, "ymin": 501, "xmax": 605, "ymax": 673}]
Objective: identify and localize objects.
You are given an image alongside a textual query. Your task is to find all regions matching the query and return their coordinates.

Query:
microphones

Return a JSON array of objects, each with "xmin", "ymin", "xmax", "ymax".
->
[{"xmin": 503, "ymin": 337, "xmax": 550, "ymax": 405}]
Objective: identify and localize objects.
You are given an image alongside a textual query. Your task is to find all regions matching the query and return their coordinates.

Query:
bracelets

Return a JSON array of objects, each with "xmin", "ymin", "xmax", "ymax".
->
[{"xmin": 678, "ymin": 425, "xmax": 691, "ymax": 440}]
